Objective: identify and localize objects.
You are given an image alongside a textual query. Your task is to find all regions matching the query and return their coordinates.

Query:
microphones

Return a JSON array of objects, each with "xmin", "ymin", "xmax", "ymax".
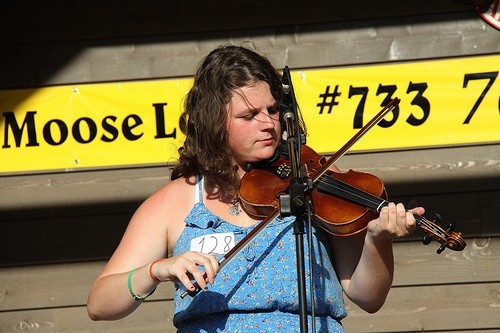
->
[{"xmin": 278, "ymin": 66, "xmax": 298, "ymax": 125}]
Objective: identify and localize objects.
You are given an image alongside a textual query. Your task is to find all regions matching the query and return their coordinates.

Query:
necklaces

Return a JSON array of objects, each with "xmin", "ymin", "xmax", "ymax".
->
[{"xmin": 215, "ymin": 178, "xmax": 242, "ymax": 215}]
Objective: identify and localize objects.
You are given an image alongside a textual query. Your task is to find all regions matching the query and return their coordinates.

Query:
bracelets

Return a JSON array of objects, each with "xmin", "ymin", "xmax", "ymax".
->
[
  {"xmin": 150, "ymin": 259, "xmax": 166, "ymax": 283},
  {"xmin": 127, "ymin": 269, "xmax": 157, "ymax": 301}
]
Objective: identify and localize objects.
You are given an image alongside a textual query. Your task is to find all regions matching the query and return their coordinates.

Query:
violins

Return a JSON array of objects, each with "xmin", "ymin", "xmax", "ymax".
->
[{"xmin": 236, "ymin": 135, "xmax": 466, "ymax": 256}]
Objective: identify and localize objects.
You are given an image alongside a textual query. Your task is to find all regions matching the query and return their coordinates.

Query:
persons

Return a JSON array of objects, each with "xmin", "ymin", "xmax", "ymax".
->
[{"xmin": 86, "ymin": 39, "xmax": 424, "ymax": 333}]
